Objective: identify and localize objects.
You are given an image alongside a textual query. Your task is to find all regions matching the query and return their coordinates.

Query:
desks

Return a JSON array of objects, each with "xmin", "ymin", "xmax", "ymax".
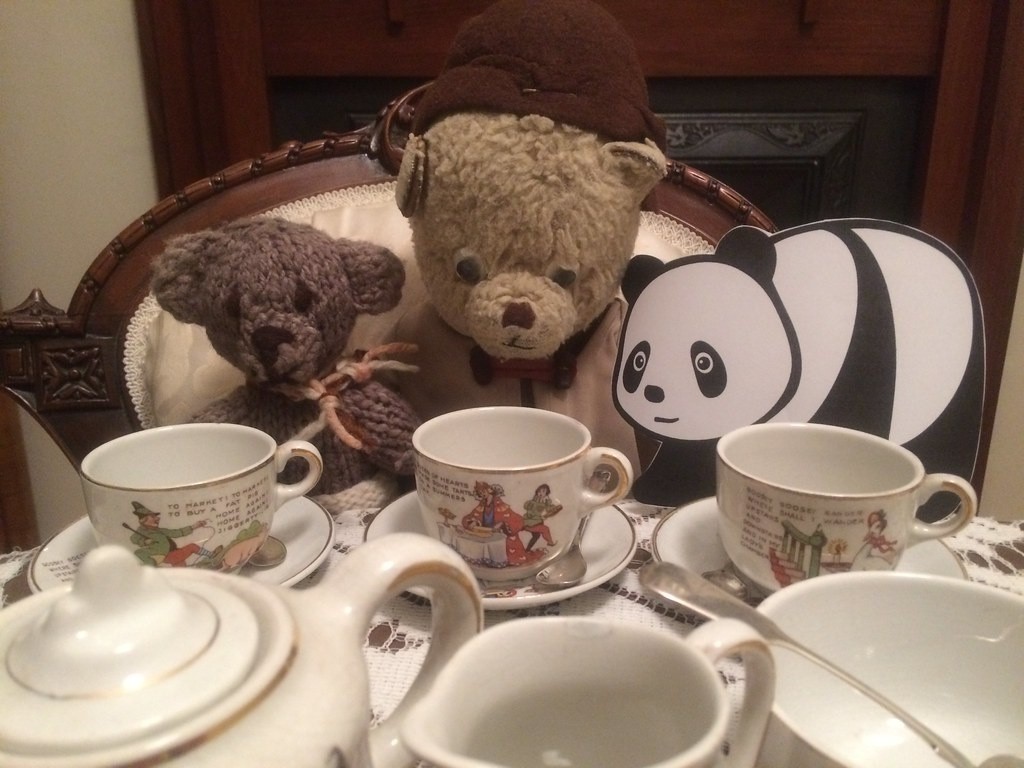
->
[{"xmin": 0, "ymin": 499, "xmax": 1024, "ymax": 768}]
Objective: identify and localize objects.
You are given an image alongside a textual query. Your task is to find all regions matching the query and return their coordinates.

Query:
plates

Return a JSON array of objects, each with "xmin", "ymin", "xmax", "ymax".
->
[
  {"xmin": 653, "ymin": 494, "xmax": 971, "ymax": 611},
  {"xmin": 362, "ymin": 490, "xmax": 639, "ymax": 611},
  {"xmin": 27, "ymin": 496, "xmax": 335, "ymax": 594}
]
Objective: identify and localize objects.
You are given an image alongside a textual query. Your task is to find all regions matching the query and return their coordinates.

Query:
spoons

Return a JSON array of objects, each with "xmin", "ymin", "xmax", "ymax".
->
[
  {"xmin": 535, "ymin": 469, "xmax": 611, "ymax": 591},
  {"xmin": 245, "ymin": 536, "xmax": 286, "ymax": 566}
]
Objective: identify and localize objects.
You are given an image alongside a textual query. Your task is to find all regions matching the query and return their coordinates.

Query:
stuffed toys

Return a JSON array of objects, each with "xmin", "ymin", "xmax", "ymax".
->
[
  {"xmin": 359, "ymin": 0, "xmax": 670, "ymax": 509},
  {"xmin": 154, "ymin": 214, "xmax": 424, "ymax": 531}
]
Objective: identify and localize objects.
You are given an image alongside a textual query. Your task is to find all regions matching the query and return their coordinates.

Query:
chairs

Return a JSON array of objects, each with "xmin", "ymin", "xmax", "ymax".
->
[{"xmin": 0, "ymin": 77, "xmax": 781, "ymax": 492}]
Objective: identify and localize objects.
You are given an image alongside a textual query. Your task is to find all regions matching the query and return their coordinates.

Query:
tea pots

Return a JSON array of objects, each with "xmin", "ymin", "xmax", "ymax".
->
[{"xmin": 0, "ymin": 535, "xmax": 482, "ymax": 768}]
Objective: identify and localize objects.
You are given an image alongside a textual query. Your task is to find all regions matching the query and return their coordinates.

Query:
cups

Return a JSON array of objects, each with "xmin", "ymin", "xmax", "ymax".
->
[
  {"xmin": 412, "ymin": 405, "xmax": 634, "ymax": 583},
  {"xmin": 80, "ymin": 423, "xmax": 323, "ymax": 576},
  {"xmin": 716, "ymin": 422, "xmax": 977, "ymax": 597}
]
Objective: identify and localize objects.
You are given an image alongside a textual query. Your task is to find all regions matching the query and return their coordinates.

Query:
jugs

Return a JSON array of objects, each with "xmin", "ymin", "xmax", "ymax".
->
[{"xmin": 401, "ymin": 614, "xmax": 776, "ymax": 768}]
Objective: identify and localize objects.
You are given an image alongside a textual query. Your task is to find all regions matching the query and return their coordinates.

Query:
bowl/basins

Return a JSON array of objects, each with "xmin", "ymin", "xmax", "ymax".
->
[{"xmin": 750, "ymin": 570, "xmax": 1024, "ymax": 767}]
